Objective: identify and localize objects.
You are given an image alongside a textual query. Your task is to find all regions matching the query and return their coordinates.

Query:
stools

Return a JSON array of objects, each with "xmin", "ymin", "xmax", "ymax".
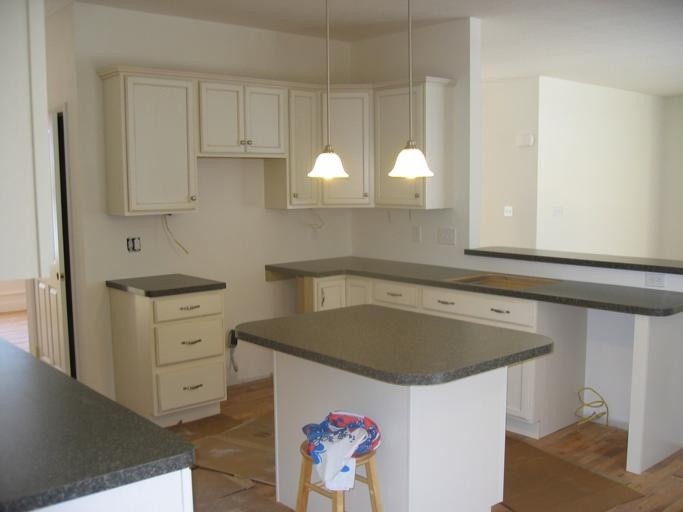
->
[{"xmin": 293, "ymin": 437, "xmax": 384, "ymax": 512}]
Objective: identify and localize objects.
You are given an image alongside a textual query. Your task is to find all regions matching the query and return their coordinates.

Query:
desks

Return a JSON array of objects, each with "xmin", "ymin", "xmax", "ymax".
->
[
  {"xmin": 1, "ymin": 336, "xmax": 197, "ymax": 512},
  {"xmin": 233, "ymin": 301, "xmax": 556, "ymax": 511}
]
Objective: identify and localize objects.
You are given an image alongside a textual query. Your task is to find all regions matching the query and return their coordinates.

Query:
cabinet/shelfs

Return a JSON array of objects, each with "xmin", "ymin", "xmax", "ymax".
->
[
  {"xmin": 0, "ymin": 1, "xmax": 55, "ymax": 281},
  {"xmin": 373, "ymin": 77, "xmax": 451, "ymax": 213},
  {"xmin": 418, "ymin": 284, "xmax": 468, "ymax": 322},
  {"xmin": 108, "ymin": 287, "xmax": 228, "ymax": 427},
  {"xmin": 345, "ymin": 272, "xmax": 371, "ymax": 308},
  {"xmin": 469, "ymin": 291, "xmax": 588, "ymax": 442},
  {"xmin": 262, "ymin": 80, "xmax": 321, "ymax": 210},
  {"xmin": 370, "ymin": 277, "xmax": 421, "ymax": 314},
  {"xmin": 296, "ymin": 274, "xmax": 344, "ymax": 315},
  {"xmin": 101, "ymin": 69, "xmax": 198, "ymax": 217},
  {"xmin": 320, "ymin": 83, "xmax": 373, "ymax": 210},
  {"xmin": 195, "ymin": 74, "xmax": 288, "ymax": 158}
]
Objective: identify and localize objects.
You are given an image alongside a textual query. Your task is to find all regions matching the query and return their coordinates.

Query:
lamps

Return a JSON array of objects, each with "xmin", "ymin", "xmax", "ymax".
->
[
  {"xmin": 386, "ymin": 0, "xmax": 435, "ymax": 182},
  {"xmin": 306, "ymin": 1, "xmax": 349, "ymax": 185}
]
